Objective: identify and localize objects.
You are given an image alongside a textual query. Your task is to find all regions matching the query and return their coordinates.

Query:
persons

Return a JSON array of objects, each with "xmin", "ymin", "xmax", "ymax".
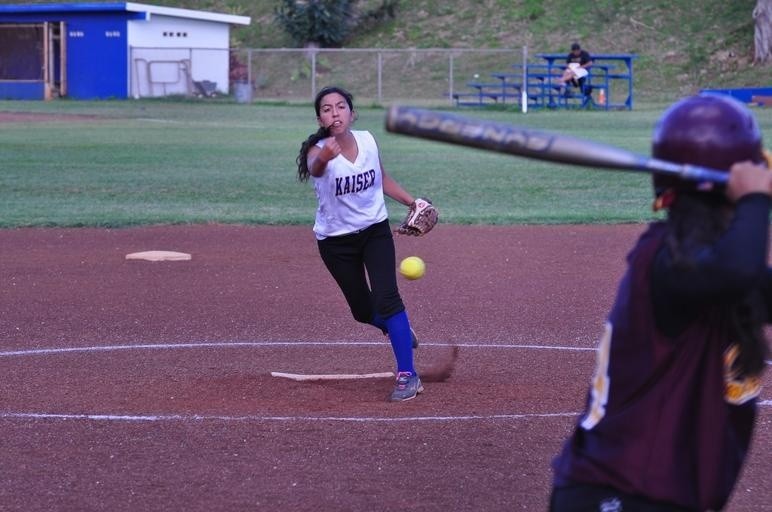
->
[
  {"xmin": 555, "ymin": 42, "xmax": 594, "ymax": 87},
  {"xmin": 546, "ymin": 91, "xmax": 772, "ymax": 512},
  {"xmin": 295, "ymin": 87, "xmax": 438, "ymax": 402}
]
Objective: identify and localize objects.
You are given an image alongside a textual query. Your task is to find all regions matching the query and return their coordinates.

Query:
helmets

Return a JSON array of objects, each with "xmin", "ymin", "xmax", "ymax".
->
[{"xmin": 647, "ymin": 92, "xmax": 770, "ymax": 209}]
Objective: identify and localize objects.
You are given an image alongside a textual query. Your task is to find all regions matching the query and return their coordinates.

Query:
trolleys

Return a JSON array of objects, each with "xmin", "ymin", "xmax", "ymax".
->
[{"xmin": 183, "ymin": 68, "xmax": 218, "ymax": 99}]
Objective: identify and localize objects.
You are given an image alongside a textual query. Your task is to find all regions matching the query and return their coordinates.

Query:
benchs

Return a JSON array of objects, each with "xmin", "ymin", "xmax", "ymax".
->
[{"xmin": 457, "ymin": 55, "xmax": 638, "ymax": 113}]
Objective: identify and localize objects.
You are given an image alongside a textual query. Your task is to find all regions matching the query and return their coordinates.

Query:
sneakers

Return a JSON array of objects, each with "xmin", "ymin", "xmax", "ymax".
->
[
  {"xmin": 389, "ymin": 370, "xmax": 424, "ymax": 400},
  {"xmin": 382, "ymin": 320, "xmax": 420, "ymax": 350}
]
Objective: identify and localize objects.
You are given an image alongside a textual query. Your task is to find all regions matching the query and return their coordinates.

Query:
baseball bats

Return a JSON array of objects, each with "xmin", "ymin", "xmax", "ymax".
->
[{"xmin": 385, "ymin": 104, "xmax": 728, "ymax": 186}]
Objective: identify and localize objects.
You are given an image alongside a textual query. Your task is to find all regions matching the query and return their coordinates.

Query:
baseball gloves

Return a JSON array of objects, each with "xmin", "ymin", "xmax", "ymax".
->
[{"xmin": 392, "ymin": 197, "xmax": 439, "ymax": 237}]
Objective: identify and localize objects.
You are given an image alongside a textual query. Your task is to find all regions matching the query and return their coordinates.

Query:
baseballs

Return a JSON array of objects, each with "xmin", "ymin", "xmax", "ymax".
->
[{"xmin": 401, "ymin": 255, "xmax": 424, "ymax": 280}]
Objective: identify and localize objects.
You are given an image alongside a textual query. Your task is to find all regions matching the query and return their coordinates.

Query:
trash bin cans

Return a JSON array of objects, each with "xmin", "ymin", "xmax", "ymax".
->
[{"xmin": 232, "ymin": 79, "xmax": 253, "ymax": 104}]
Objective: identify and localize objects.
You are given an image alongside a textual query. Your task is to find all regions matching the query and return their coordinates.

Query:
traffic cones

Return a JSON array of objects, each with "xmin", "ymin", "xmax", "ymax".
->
[{"xmin": 595, "ymin": 87, "xmax": 606, "ymax": 105}]
